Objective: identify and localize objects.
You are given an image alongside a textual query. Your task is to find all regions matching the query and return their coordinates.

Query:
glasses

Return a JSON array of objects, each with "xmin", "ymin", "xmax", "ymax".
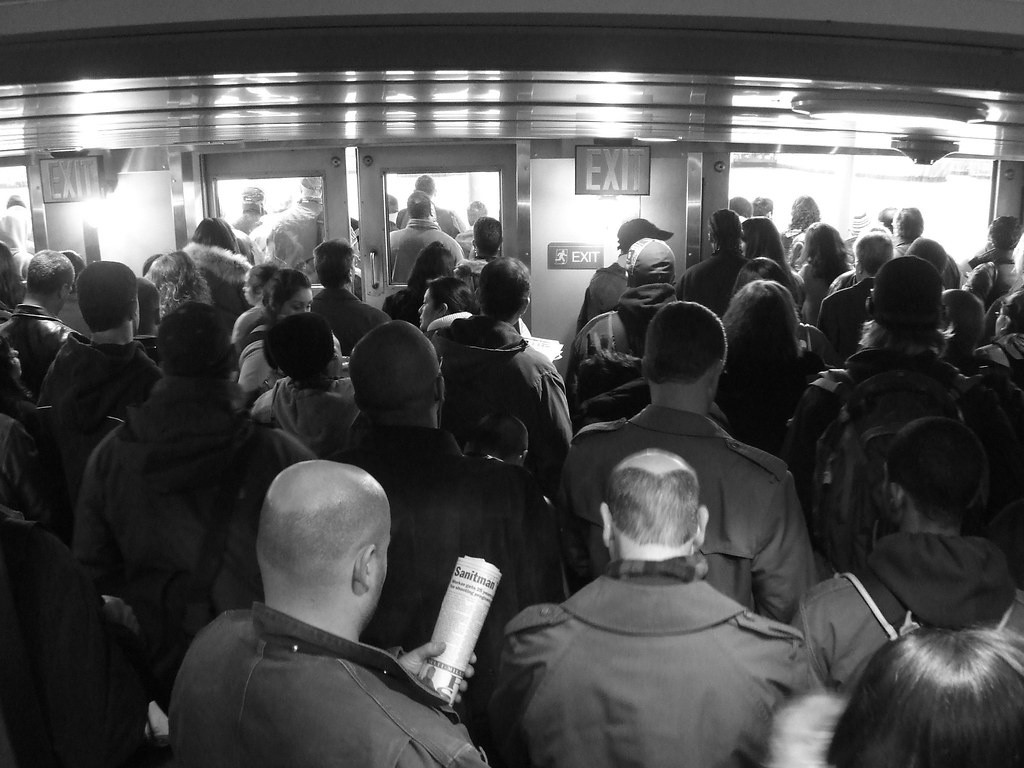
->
[{"xmin": 994, "ymin": 311, "xmax": 1007, "ymax": 318}]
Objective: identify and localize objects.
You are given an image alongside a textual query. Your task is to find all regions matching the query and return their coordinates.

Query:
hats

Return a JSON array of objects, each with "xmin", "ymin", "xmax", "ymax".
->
[
  {"xmin": 618, "ymin": 238, "xmax": 676, "ymax": 274},
  {"xmin": 615, "ymin": 219, "xmax": 673, "ymax": 247},
  {"xmin": 302, "ymin": 177, "xmax": 323, "ymax": 189},
  {"xmin": 265, "ymin": 312, "xmax": 334, "ymax": 380},
  {"xmin": 849, "ymin": 213, "xmax": 873, "ymax": 234},
  {"xmin": 243, "ymin": 186, "xmax": 265, "ymax": 213},
  {"xmin": 870, "ymin": 256, "xmax": 943, "ymax": 329},
  {"xmin": 156, "ymin": 302, "xmax": 232, "ymax": 376}
]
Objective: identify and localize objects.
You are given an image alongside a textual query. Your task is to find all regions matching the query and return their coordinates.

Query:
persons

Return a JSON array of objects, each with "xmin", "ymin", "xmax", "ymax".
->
[
  {"xmin": 420, "ymin": 667, "xmax": 436, "ymax": 689},
  {"xmin": 555, "ymin": 301, "xmax": 819, "ymax": 623},
  {"xmin": 0, "ymin": 176, "xmax": 1024, "ymax": 768}
]
